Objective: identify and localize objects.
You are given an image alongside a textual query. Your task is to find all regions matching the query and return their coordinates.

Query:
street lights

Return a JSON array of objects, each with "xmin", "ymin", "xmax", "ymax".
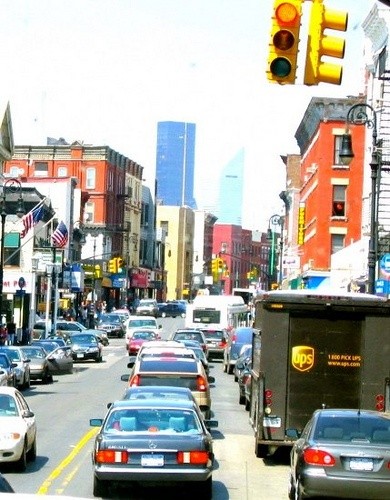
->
[
  {"xmin": 0, "ymin": 177, "xmax": 26, "ymax": 307},
  {"xmin": 44, "ymin": 263, "xmax": 63, "ymax": 339},
  {"xmin": 266, "ymin": 213, "xmax": 287, "ymax": 290},
  {"xmin": 340, "ymin": 101, "xmax": 382, "ymax": 295}
]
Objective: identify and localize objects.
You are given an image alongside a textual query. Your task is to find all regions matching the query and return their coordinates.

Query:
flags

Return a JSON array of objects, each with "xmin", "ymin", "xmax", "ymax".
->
[
  {"xmin": 51, "ymin": 221, "xmax": 68, "ymax": 247},
  {"xmin": 20, "ymin": 204, "xmax": 44, "ymax": 238}
]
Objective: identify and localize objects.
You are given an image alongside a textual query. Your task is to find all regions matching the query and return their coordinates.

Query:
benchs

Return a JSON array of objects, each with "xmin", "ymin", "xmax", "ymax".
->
[
  {"xmin": 323, "ymin": 427, "xmax": 390, "ymax": 443},
  {"xmin": 120, "ymin": 417, "xmax": 188, "ymax": 432}
]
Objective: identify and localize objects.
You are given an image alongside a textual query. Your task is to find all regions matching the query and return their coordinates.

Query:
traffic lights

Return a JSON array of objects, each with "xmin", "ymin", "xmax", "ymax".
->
[
  {"xmin": 247, "ymin": 273, "xmax": 251, "ymax": 278},
  {"xmin": 212, "ymin": 258, "xmax": 223, "ymax": 274},
  {"xmin": 108, "ymin": 257, "xmax": 124, "ymax": 273},
  {"xmin": 264, "ymin": 0, "xmax": 305, "ymax": 84},
  {"xmin": 301, "ymin": 1, "xmax": 349, "ymax": 86}
]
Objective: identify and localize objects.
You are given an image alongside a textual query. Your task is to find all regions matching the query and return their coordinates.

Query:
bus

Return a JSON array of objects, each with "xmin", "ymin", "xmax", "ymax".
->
[
  {"xmin": 185, "ymin": 294, "xmax": 248, "ymax": 332},
  {"xmin": 232, "ymin": 287, "xmax": 266, "ymax": 305}
]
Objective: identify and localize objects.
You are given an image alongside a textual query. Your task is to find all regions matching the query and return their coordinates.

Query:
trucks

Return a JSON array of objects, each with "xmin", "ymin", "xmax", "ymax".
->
[{"xmin": 249, "ymin": 290, "xmax": 390, "ymax": 456}]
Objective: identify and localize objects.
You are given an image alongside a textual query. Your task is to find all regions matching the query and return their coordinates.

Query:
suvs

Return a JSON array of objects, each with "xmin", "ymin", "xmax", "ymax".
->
[
  {"xmin": 95, "ymin": 308, "xmax": 162, "ymax": 340},
  {"xmin": 31, "ymin": 319, "xmax": 109, "ymax": 346},
  {"xmin": 136, "ymin": 298, "xmax": 157, "ymax": 318}
]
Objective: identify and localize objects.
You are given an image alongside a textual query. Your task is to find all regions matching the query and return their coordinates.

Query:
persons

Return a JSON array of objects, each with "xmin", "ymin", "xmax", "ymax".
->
[
  {"xmin": 6, "ymin": 321, "xmax": 16, "ymax": 345},
  {"xmin": 66, "ymin": 305, "xmax": 88, "ymax": 325}
]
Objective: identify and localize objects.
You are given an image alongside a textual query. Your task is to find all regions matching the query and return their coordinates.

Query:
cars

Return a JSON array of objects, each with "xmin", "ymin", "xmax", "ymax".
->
[
  {"xmin": 90, "ymin": 400, "xmax": 218, "ymax": 483},
  {"xmin": 171, "ymin": 327, "xmax": 226, "ymax": 359},
  {"xmin": 157, "ymin": 300, "xmax": 186, "ymax": 318},
  {"xmin": 291, "ymin": 403, "xmax": 390, "ymax": 500},
  {"xmin": 200, "ymin": 329, "xmax": 227, "ymax": 360},
  {"xmin": 0, "ymin": 332, "xmax": 103, "ymax": 390},
  {"xmin": 126, "ymin": 330, "xmax": 161, "ymax": 356},
  {"xmin": 121, "ymin": 340, "xmax": 216, "ymax": 416},
  {"xmin": 0, "ymin": 386, "xmax": 38, "ymax": 494},
  {"xmin": 223, "ymin": 326, "xmax": 254, "ymax": 410}
]
